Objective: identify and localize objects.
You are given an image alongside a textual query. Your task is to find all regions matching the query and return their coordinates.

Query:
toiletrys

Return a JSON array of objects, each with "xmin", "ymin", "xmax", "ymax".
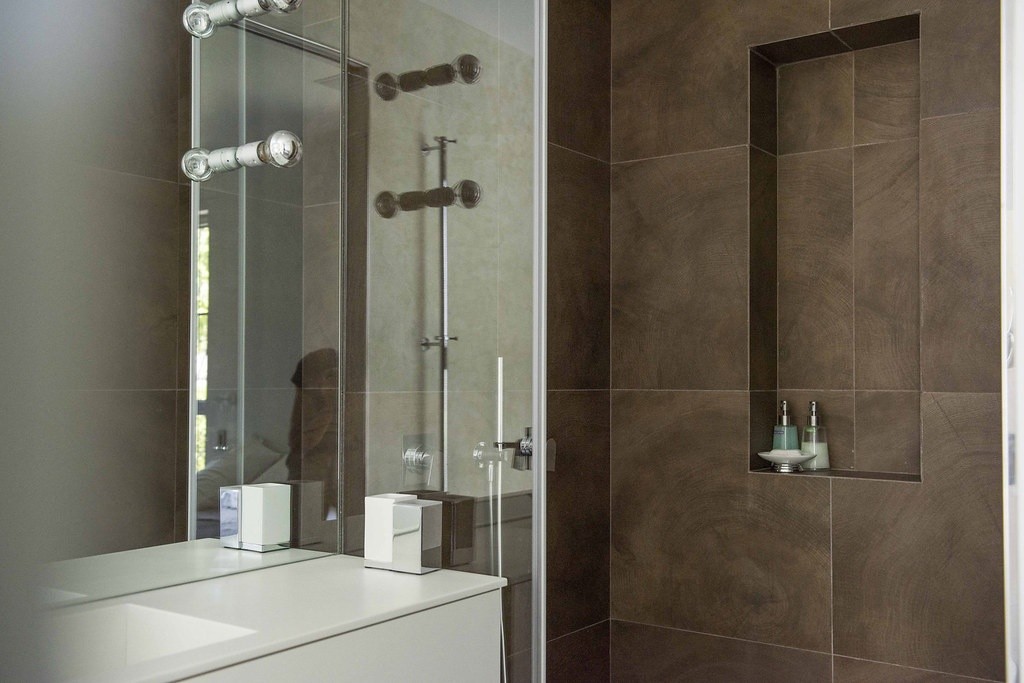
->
[
  {"xmin": 772, "ymin": 400, "xmax": 800, "ymax": 450},
  {"xmin": 801, "ymin": 399, "xmax": 832, "ymax": 472}
]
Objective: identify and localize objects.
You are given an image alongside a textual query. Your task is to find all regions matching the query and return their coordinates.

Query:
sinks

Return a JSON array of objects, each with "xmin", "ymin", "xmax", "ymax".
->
[
  {"xmin": 2, "ymin": 585, "xmax": 88, "ymax": 619},
  {"xmin": 0, "ymin": 604, "xmax": 260, "ymax": 683}
]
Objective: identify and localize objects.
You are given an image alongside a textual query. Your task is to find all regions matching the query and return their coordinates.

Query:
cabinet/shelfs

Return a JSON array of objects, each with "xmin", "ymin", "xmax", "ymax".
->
[{"xmin": 0, "ymin": 537, "xmax": 508, "ymax": 683}]
